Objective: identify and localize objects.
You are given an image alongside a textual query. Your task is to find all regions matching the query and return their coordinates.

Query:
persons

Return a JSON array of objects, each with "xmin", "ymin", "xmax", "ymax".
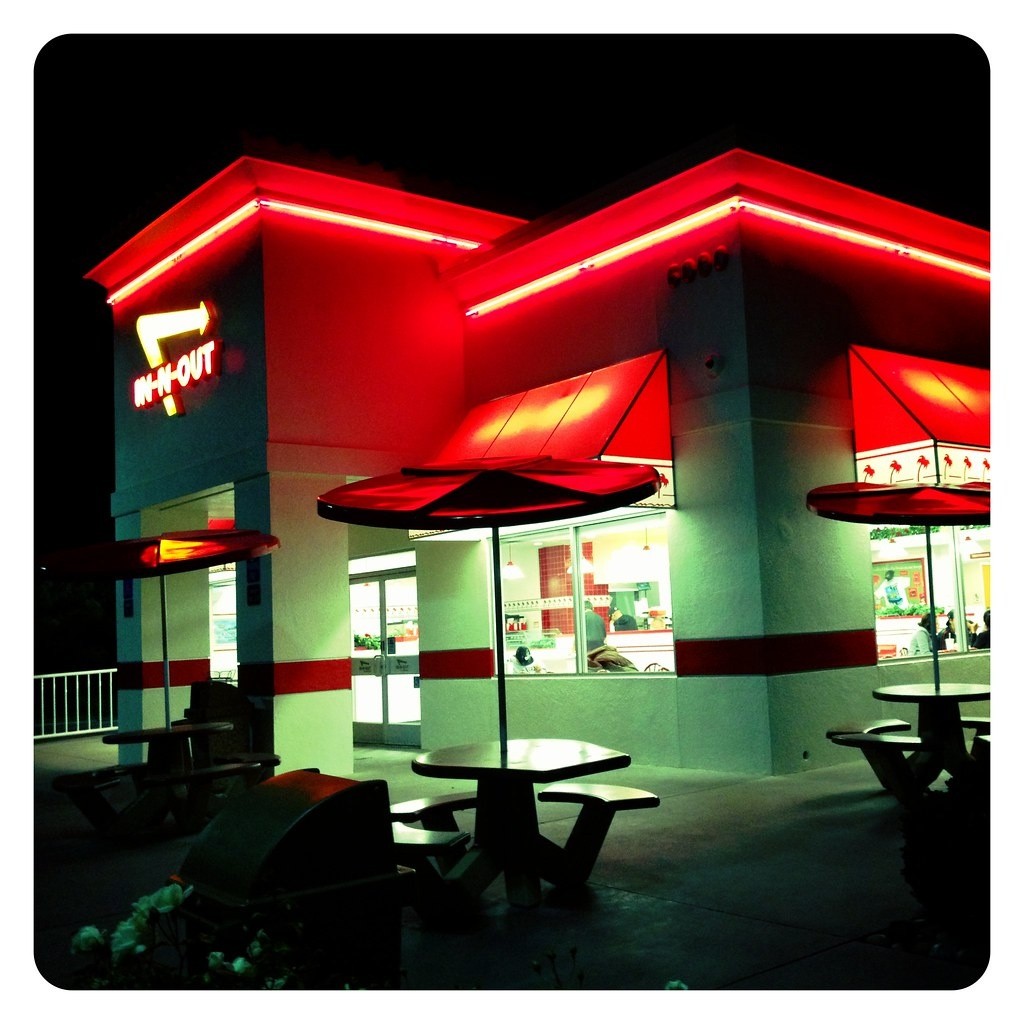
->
[
  {"xmin": 910, "ymin": 608, "xmax": 989, "ymax": 653},
  {"xmin": 583, "ymin": 601, "xmax": 606, "ymax": 652},
  {"xmin": 608, "ymin": 607, "xmax": 639, "ymax": 631}
]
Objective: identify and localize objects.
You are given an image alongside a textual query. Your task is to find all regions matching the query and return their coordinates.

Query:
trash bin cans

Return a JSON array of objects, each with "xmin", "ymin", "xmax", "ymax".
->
[
  {"xmin": 166, "ymin": 767, "xmax": 402, "ymax": 991},
  {"xmin": 184, "ymin": 681, "xmax": 253, "ymax": 754}
]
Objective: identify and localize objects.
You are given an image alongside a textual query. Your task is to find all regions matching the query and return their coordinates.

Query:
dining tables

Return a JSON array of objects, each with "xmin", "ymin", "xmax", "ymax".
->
[
  {"xmin": 100, "ymin": 721, "xmax": 235, "ymax": 810},
  {"xmin": 412, "ymin": 736, "xmax": 634, "ymax": 905},
  {"xmin": 872, "ymin": 681, "xmax": 989, "ymax": 788}
]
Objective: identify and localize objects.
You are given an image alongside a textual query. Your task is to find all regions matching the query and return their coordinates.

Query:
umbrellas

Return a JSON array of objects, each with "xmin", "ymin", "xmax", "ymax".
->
[
  {"xmin": 50, "ymin": 531, "xmax": 282, "ymax": 731},
  {"xmin": 808, "ymin": 481, "xmax": 990, "ymax": 689},
  {"xmin": 317, "ymin": 454, "xmax": 660, "ymax": 750}
]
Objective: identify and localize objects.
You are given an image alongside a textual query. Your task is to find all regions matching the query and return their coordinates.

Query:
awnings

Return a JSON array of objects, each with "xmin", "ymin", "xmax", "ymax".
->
[
  {"xmin": 846, "ymin": 345, "xmax": 989, "ymax": 486},
  {"xmin": 406, "ymin": 349, "xmax": 677, "ymax": 545}
]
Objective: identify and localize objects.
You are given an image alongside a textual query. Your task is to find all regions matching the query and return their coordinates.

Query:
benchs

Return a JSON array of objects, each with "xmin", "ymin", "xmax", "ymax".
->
[
  {"xmin": 538, "ymin": 780, "xmax": 658, "ymax": 896},
  {"xmin": 163, "ymin": 760, "xmax": 262, "ymax": 836},
  {"xmin": 957, "ymin": 714, "xmax": 990, "ymax": 803},
  {"xmin": 390, "ymin": 786, "xmax": 486, "ymax": 899},
  {"xmin": 58, "ymin": 760, "xmax": 155, "ymax": 830},
  {"xmin": 829, "ymin": 717, "xmax": 932, "ymax": 805},
  {"xmin": 214, "ymin": 749, "xmax": 281, "ymax": 802}
]
